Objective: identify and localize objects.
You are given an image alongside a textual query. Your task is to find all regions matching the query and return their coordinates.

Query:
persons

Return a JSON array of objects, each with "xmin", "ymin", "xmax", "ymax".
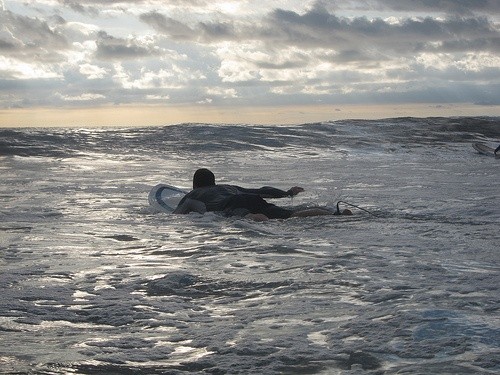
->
[{"xmin": 171, "ymin": 168, "xmax": 354, "ymax": 240}]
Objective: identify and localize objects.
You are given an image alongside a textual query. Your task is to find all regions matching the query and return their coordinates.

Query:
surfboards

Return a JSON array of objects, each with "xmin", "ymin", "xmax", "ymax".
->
[
  {"xmin": 147, "ymin": 182, "xmax": 369, "ymax": 227},
  {"xmin": 472, "ymin": 142, "xmax": 500, "ymax": 156}
]
